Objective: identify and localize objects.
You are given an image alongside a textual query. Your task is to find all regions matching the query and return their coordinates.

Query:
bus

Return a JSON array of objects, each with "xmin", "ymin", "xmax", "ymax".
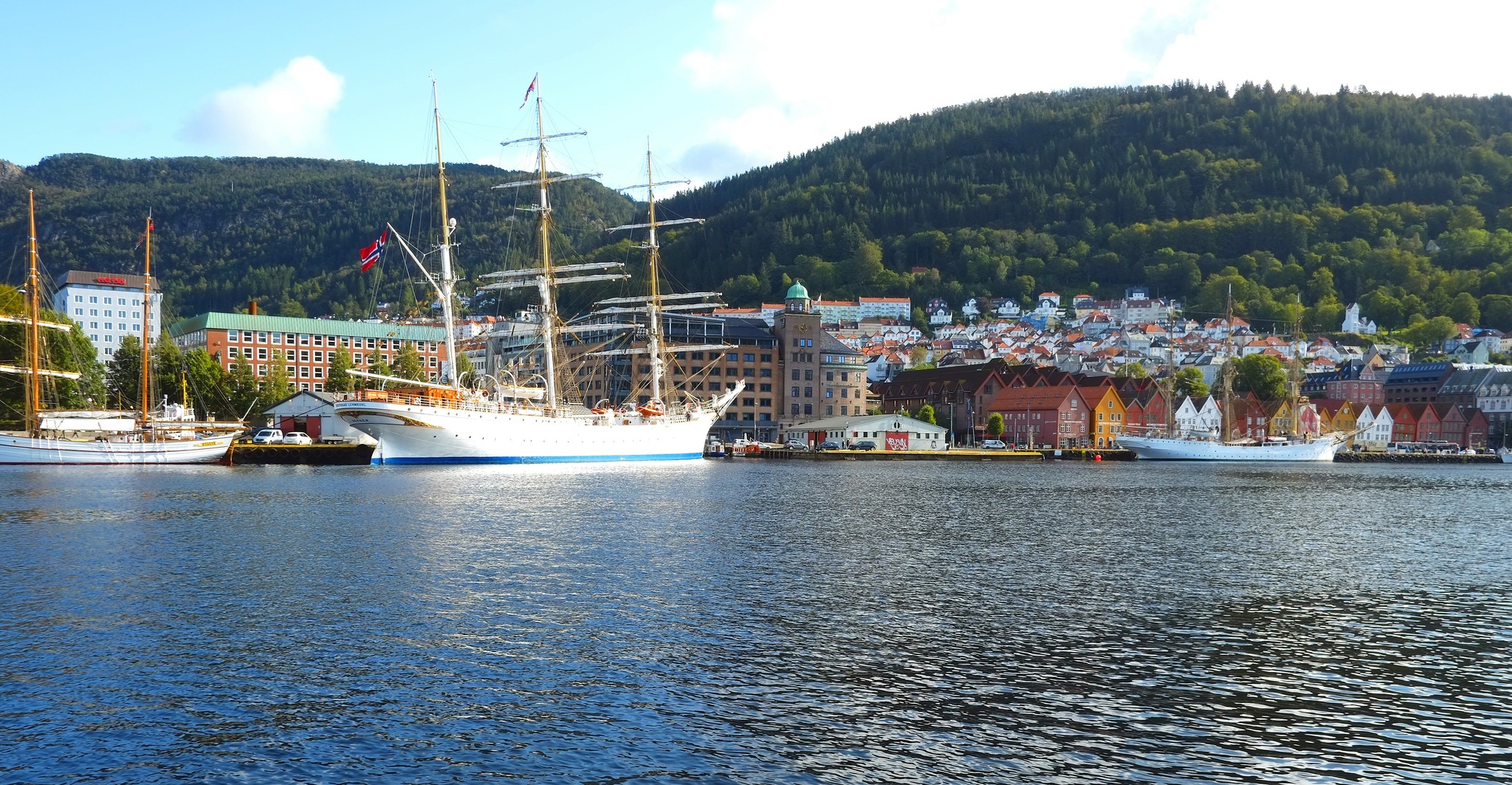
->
[{"xmin": 1387, "ymin": 440, "xmax": 1460, "ymax": 455}]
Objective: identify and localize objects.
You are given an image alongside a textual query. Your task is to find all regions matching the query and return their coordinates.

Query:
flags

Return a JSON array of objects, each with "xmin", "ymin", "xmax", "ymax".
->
[
  {"xmin": 134, "ymin": 221, "xmax": 154, "ymax": 250},
  {"xmin": 360, "ymin": 231, "xmax": 387, "ymax": 273}
]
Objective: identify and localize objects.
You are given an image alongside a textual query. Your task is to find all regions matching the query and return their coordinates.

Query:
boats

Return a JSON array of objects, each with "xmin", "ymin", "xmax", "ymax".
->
[
  {"xmin": 590, "ymin": 407, "xmax": 625, "ymax": 415},
  {"xmin": 745, "ymin": 444, "xmax": 761, "ymax": 458},
  {"xmin": 1501, "ymin": 454, "xmax": 1512, "ymax": 463},
  {"xmin": 703, "ymin": 435, "xmax": 725, "ymax": 457},
  {"xmin": 635, "ymin": 400, "xmax": 664, "ymax": 417},
  {"xmin": 731, "ymin": 447, "xmax": 746, "ymax": 457},
  {"xmin": 493, "ymin": 383, "xmax": 545, "ymax": 400}
]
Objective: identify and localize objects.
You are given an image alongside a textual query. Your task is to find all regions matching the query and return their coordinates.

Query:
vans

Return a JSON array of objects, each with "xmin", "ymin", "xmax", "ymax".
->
[
  {"xmin": 731, "ymin": 439, "xmax": 751, "ymax": 447},
  {"xmin": 981, "ymin": 440, "xmax": 1006, "ymax": 449},
  {"xmin": 253, "ymin": 429, "xmax": 284, "ymax": 445}
]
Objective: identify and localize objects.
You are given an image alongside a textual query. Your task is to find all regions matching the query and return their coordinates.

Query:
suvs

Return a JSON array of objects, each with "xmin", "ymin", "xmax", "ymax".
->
[
  {"xmin": 849, "ymin": 441, "xmax": 877, "ymax": 451},
  {"xmin": 815, "ymin": 441, "xmax": 840, "ymax": 451},
  {"xmin": 783, "ymin": 438, "xmax": 809, "ymax": 450}
]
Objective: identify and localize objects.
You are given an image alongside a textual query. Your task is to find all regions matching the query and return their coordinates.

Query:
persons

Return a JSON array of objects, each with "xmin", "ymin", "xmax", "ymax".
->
[
  {"xmin": 841, "ymin": 440, "xmax": 846, "ymax": 450},
  {"xmin": 1465, "ymin": 448, "xmax": 1468, "ymax": 454},
  {"xmin": 1011, "ymin": 443, "xmax": 1014, "ymax": 453},
  {"xmin": 957, "ymin": 439, "xmax": 961, "ymax": 448},
  {"xmin": 686, "ymin": 413, "xmax": 690, "ymax": 421},
  {"xmin": 1025, "ymin": 444, "xmax": 1028, "ymax": 452},
  {"xmin": 775, "ymin": 437, "xmax": 778, "ymax": 444},
  {"xmin": 1039, "ymin": 442, "xmax": 1045, "ymax": 449},
  {"xmin": 1456, "ymin": 444, "xmax": 1460, "ymax": 454},
  {"xmin": 846, "ymin": 439, "xmax": 851, "ymax": 450}
]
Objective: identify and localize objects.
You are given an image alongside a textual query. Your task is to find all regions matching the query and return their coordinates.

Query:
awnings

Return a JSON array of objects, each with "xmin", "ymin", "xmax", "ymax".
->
[{"xmin": 977, "ymin": 434, "xmax": 1007, "ymax": 437}]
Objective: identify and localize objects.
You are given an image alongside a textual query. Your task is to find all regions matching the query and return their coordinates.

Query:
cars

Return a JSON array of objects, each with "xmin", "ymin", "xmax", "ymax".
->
[
  {"xmin": 752, "ymin": 441, "xmax": 772, "ymax": 450},
  {"xmin": 283, "ymin": 432, "xmax": 313, "ymax": 446},
  {"xmin": 1460, "ymin": 449, "xmax": 1476, "ymax": 455},
  {"xmin": 1484, "ymin": 449, "xmax": 1495, "ymax": 454}
]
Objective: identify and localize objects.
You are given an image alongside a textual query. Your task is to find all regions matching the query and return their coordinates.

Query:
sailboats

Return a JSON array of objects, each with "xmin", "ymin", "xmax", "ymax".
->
[
  {"xmin": 369, "ymin": 68, "xmax": 745, "ymax": 467},
  {"xmin": 1, "ymin": 187, "xmax": 249, "ymax": 465},
  {"xmin": 1114, "ymin": 281, "xmax": 1376, "ymax": 462}
]
